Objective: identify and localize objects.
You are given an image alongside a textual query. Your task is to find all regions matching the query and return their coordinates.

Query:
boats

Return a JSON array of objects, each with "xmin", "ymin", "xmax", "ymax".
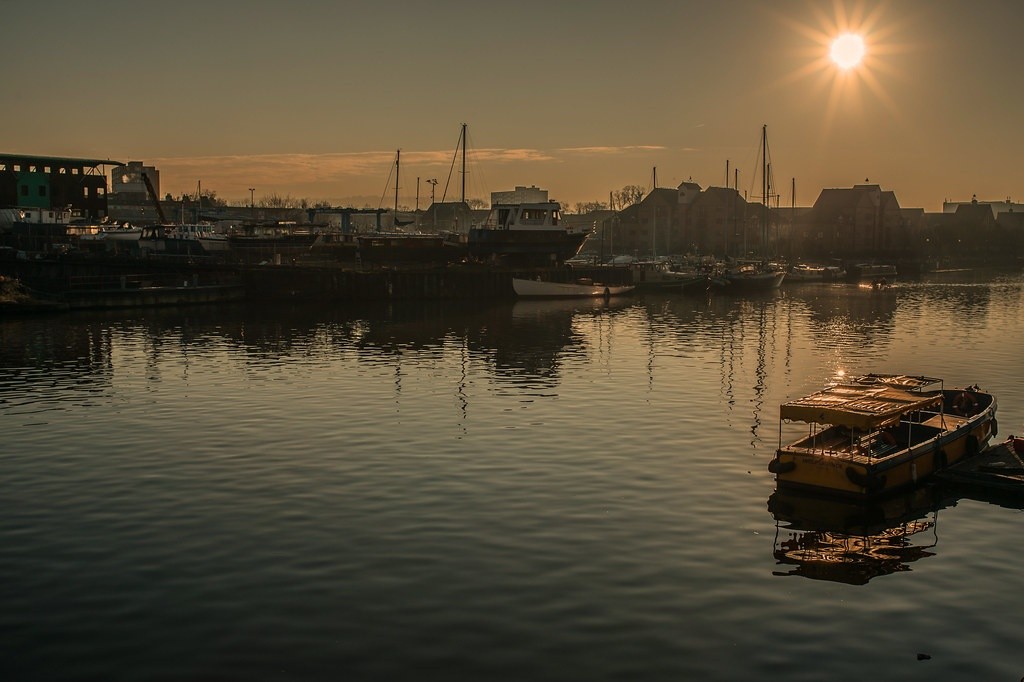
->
[
  {"xmin": 766, "ymin": 440, "xmax": 989, "ymax": 586},
  {"xmin": 766, "ymin": 371, "xmax": 999, "ymax": 500},
  {"xmin": 510, "ymin": 271, "xmax": 638, "ymax": 298},
  {"xmin": 64, "ymin": 121, "xmax": 900, "ymax": 290}
]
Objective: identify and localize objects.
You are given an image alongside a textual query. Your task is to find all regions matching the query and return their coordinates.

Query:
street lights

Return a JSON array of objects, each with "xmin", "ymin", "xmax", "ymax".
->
[
  {"xmin": 249, "ymin": 188, "xmax": 255, "ymax": 218},
  {"xmin": 426, "ymin": 179, "xmax": 439, "ymax": 236}
]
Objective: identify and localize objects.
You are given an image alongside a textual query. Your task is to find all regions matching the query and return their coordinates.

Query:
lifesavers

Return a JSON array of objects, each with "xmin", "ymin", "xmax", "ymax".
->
[
  {"xmin": 952, "ymin": 392, "xmax": 977, "ymax": 417},
  {"xmin": 965, "ymin": 435, "xmax": 979, "ymax": 456}
]
